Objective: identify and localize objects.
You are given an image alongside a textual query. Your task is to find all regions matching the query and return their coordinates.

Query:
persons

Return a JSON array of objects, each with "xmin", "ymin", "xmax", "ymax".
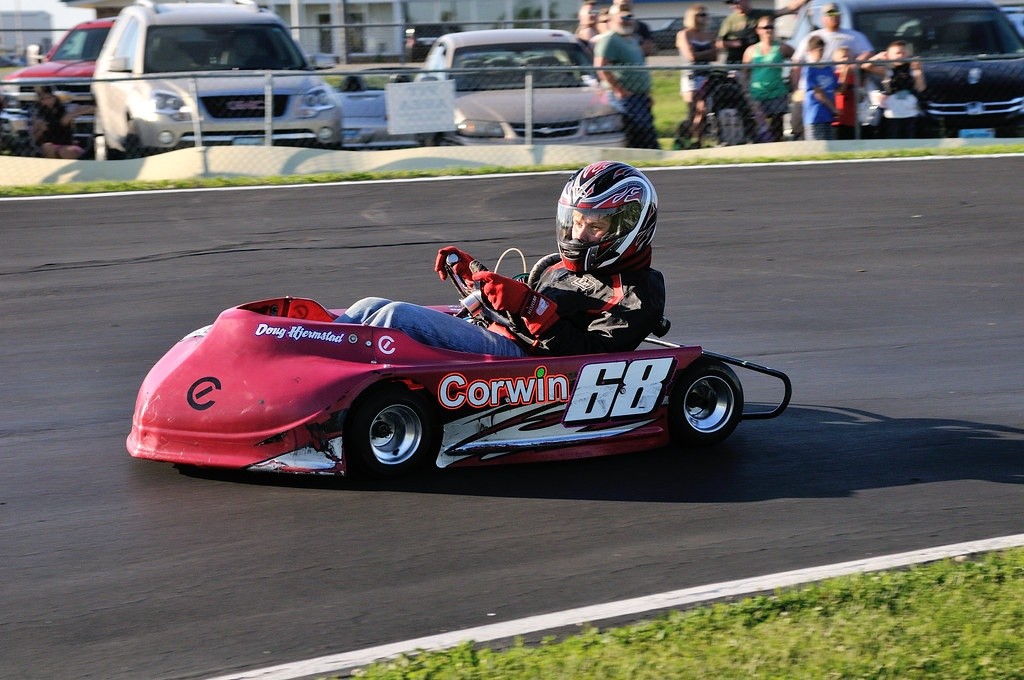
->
[
  {"xmin": 675, "ymin": 0, "xmax": 928, "ymax": 148},
  {"xmin": 575, "ymin": 0, "xmax": 661, "ymax": 149},
  {"xmin": 35, "ymin": 86, "xmax": 96, "ymax": 159},
  {"xmin": 333, "ymin": 161, "xmax": 666, "ymax": 357}
]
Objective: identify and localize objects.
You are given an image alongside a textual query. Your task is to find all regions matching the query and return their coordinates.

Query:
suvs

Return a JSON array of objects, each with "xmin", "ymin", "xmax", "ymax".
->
[
  {"xmin": 90, "ymin": 1, "xmax": 345, "ymax": 154},
  {"xmin": 1, "ymin": 15, "xmax": 122, "ymax": 151}
]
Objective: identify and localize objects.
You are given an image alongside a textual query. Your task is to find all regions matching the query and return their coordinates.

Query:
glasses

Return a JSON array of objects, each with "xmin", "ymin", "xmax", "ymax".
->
[
  {"xmin": 756, "ymin": 26, "xmax": 774, "ymax": 29},
  {"xmin": 696, "ymin": 13, "xmax": 707, "ymax": 18}
]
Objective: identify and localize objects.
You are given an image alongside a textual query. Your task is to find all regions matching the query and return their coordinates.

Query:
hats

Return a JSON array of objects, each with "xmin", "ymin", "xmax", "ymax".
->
[
  {"xmin": 608, "ymin": 2, "xmax": 635, "ymax": 18},
  {"xmin": 822, "ymin": 3, "xmax": 842, "ymax": 16}
]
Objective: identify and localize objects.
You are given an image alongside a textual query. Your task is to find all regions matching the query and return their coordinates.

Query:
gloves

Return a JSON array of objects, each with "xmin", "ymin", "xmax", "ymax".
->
[
  {"xmin": 435, "ymin": 247, "xmax": 490, "ymax": 288},
  {"xmin": 471, "ymin": 271, "xmax": 560, "ymax": 338}
]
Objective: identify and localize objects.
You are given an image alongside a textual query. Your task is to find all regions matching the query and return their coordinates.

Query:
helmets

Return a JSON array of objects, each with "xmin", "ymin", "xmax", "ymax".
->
[{"xmin": 556, "ymin": 161, "xmax": 658, "ymax": 273}]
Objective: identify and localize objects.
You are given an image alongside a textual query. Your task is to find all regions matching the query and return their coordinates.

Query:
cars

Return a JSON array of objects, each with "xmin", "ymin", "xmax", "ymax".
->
[
  {"xmin": 332, "ymin": 75, "xmax": 425, "ymax": 151},
  {"xmin": 782, "ymin": 0, "xmax": 1024, "ymax": 138},
  {"xmin": 413, "ymin": 29, "xmax": 630, "ymax": 152}
]
